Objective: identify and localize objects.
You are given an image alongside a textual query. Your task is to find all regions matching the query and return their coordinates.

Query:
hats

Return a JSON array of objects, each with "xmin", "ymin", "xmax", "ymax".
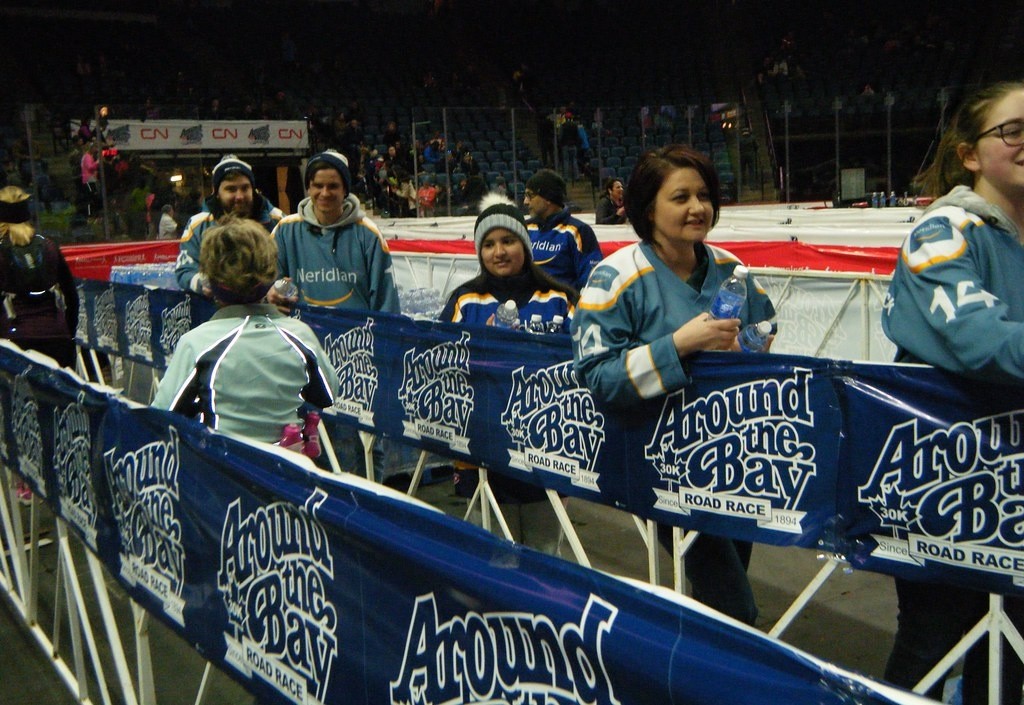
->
[
  {"xmin": 525, "ymin": 169, "xmax": 568, "ymax": 206},
  {"xmin": 305, "ymin": 148, "xmax": 353, "ymax": 198},
  {"xmin": 208, "ymin": 153, "xmax": 257, "ymax": 197},
  {"xmin": 474, "ymin": 190, "xmax": 534, "ymax": 264}
]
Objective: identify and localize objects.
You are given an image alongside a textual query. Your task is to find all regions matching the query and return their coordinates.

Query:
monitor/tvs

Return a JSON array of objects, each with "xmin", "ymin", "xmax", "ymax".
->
[{"xmin": 837, "ymin": 166, "xmax": 867, "ymax": 204}]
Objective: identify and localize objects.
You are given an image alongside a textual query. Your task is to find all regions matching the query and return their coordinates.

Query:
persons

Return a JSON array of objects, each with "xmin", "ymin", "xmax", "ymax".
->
[
  {"xmin": 570, "ymin": 143, "xmax": 778, "ymax": 626},
  {"xmin": 0, "ymin": 186, "xmax": 79, "ymax": 506},
  {"xmin": 41, "ymin": 53, "xmax": 290, "ymax": 238},
  {"xmin": 0, "ymin": 135, "xmax": 65, "ymax": 215},
  {"xmin": 757, "ymin": 50, "xmax": 807, "ymax": 104},
  {"xmin": 147, "ymin": 209, "xmax": 341, "ymax": 456},
  {"xmin": 884, "ymin": 81, "xmax": 1024, "ymax": 705},
  {"xmin": 174, "ymin": 153, "xmax": 289, "ymax": 296},
  {"xmin": 289, "ymin": 91, "xmax": 515, "ymax": 218},
  {"xmin": 596, "ymin": 178, "xmax": 628, "ymax": 224},
  {"xmin": 533, "ymin": 97, "xmax": 590, "ymax": 183},
  {"xmin": 421, "ymin": 60, "xmax": 536, "ymax": 109},
  {"xmin": 263, "ymin": 149, "xmax": 401, "ymax": 480},
  {"xmin": 523, "ymin": 169, "xmax": 603, "ymax": 294},
  {"xmin": 436, "ymin": 191, "xmax": 582, "ymax": 558}
]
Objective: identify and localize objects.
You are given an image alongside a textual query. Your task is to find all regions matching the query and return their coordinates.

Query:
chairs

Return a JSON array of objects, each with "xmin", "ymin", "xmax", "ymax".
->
[{"xmin": 8, "ymin": 7, "xmax": 1003, "ymax": 215}]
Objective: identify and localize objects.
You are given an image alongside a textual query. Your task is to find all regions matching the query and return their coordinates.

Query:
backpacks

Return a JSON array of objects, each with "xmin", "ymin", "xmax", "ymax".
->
[{"xmin": 0, "ymin": 237, "xmax": 74, "ymax": 359}]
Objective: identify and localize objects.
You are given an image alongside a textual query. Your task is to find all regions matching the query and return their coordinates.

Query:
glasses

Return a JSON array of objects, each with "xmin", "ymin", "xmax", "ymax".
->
[{"xmin": 972, "ymin": 119, "xmax": 1024, "ymax": 147}]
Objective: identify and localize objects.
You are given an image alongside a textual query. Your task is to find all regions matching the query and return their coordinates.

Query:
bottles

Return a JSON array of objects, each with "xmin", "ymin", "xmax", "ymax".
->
[
  {"xmin": 879, "ymin": 192, "xmax": 886, "ymax": 207},
  {"xmin": 279, "ymin": 422, "xmax": 307, "ymax": 457},
  {"xmin": 303, "ymin": 410, "xmax": 322, "ymax": 459},
  {"xmin": 274, "ymin": 279, "xmax": 298, "ymax": 299},
  {"xmin": 546, "ymin": 314, "xmax": 564, "ymax": 335},
  {"xmin": 492, "ymin": 299, "xmax": 519, "ymax": 330},
  {"xmin": 705, "ymin": 264, "xmax": 749, "ymax": 321},
  {"xmin": 110, "ymin": 262, "xmax": 184, "ymax": 292},
  {"xmin": 397, "ymin": 287, "xmax": 447, "ymax": 321},
  {"xmin": 889, "ymin": 191, "xmax": 896, "ymax": 207},
  {"xmin": 727, "ymin": 320, "xmax": 773, "ymax": 354},
  {"xmin": 871, "ymin": 192, "xmax": 878, "ymax": 208},
  {"xmin": 529, "ymin": 314, "xmax": 545, "ymax": 336}
]
